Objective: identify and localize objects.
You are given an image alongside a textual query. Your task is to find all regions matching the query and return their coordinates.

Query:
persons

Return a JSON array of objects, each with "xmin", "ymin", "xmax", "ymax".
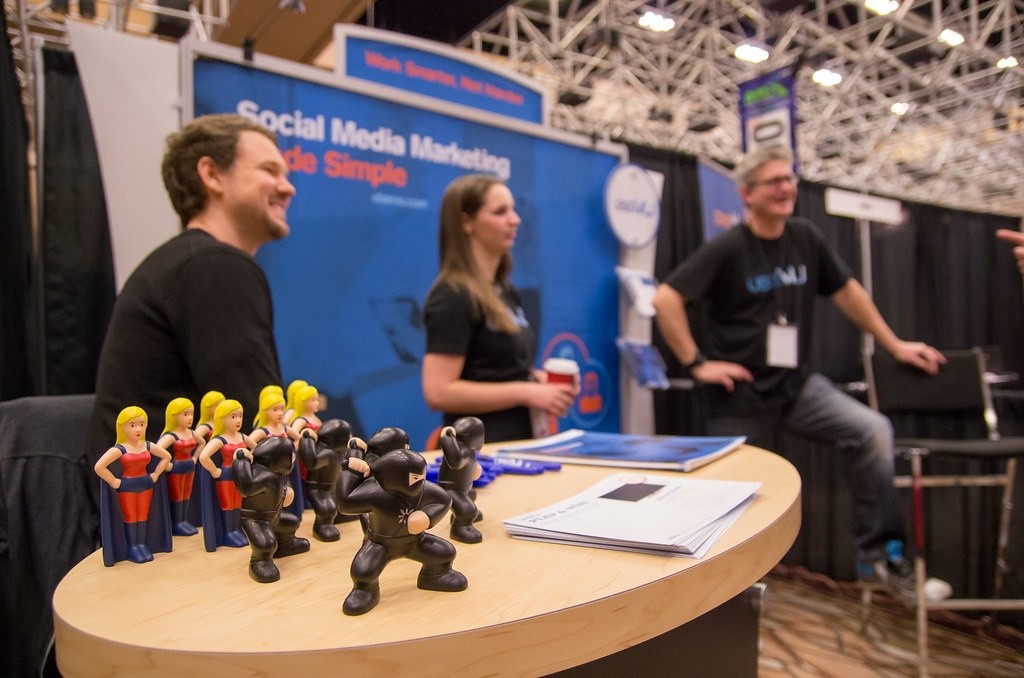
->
[
  {"xmin": 93, "ymin": 379, "xmax": 486, "ymax": 616},
  {"xmin": 652, "ymin": 142, "xmax": 954, "ymax": 603},
  {"xmin": 420, "ymin": 173, "xmax": 583, "ymax": 448},
  {"xmin": 90, "ymin": 108, "xmax": 297, "ymax": 553},
  {"xmin": 995, "ymin": 228, "xmax": 1024, "ymax": 275}
]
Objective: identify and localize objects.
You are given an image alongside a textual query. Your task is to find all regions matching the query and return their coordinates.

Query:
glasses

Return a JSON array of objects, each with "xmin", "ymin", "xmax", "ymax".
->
[{"xmin": 746, "ymin": 174, "xmax": 799, "ymax": 187}]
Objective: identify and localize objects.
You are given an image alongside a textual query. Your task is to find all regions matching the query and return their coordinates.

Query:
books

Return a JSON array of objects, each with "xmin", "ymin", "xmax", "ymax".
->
[{"xmin": 496, "ymin": 428, "xmax": 747, "ymax": 474}]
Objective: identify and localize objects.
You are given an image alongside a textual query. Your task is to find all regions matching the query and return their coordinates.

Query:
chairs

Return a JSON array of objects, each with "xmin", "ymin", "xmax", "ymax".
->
[{"xmin": 859, "ymin": 345, "xmax": 1024, "ymax": 677}]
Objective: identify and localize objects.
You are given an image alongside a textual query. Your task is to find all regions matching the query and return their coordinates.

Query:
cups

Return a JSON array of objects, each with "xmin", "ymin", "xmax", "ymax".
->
[{"xmin": 543, "ymin": 356, "xmax": 579, "ymax": 388}]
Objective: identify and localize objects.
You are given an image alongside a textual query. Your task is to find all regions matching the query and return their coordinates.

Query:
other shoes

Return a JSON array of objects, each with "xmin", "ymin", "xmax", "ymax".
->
[{"xmin": 856, "ymin": 555, "xmax": 951, "ymax": 605}]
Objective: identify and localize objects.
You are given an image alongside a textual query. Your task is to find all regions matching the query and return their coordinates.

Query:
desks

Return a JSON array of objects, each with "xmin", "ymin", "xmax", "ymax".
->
[{"xmin": 51, "ymin": 435, "xmax": 803, "ymax": 678}]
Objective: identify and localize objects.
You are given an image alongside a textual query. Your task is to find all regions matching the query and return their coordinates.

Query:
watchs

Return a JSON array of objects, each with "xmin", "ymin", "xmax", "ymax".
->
[{"xmin": 685, "ymin": 350, "xmax": 706, "ymax": 372}]
